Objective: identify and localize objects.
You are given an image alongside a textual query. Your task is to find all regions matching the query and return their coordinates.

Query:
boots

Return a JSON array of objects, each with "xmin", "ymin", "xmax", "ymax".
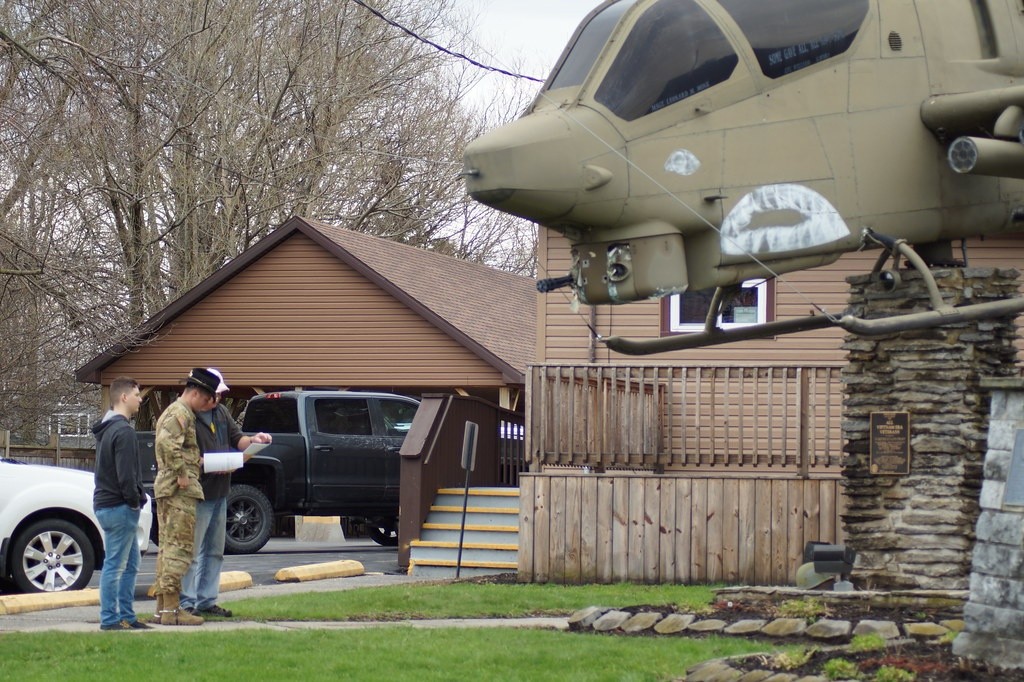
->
[
  {"xmin": 161, "ymin": 593, "xmax": 203, "ymax": 626},
  {"xmin": 152, "ymin": 594, "xmax": 164, "ymax": 623}
]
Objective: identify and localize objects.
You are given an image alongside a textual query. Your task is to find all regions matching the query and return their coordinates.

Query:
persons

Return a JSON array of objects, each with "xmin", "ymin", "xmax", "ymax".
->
[
  {"xmin": 91, "ymin": 377, "xmax": 150, "ymax": 630},
  {"xmin": 154, "ymin": 368, "xmax": 220, "ymax": 625},
  {"xmin": 178, "ymin": 369, "xmax": 272, "ymax": 617}
]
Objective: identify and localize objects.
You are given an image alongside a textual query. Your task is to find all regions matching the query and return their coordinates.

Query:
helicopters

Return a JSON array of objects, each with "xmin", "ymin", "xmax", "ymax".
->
[{"xmin": 459, "ymin": 0, "xmax": 1024, "ymax": 354}]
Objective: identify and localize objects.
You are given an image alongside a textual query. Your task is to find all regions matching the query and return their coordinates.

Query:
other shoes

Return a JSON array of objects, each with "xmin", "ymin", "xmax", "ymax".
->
[
  {"xmin": 100, "ymin": 620, "xmax": 134, "ymax": 631},
  {"xmin": 208, "ymin": 605, "xmax": 232, "ymax": 617},
  {"xmin": 185, "ymin": 608, "xmax": 201, "ymax": 616},
  {"xmin": 132, "ymin": 621, "xmax": 149, "ymax": 629}
]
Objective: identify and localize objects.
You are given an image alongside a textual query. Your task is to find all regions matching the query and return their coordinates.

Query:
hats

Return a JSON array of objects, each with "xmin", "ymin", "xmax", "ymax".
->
[
  {"xmin": 179, "ymin": 368, "xmax": 221, "ymax": 402},
  {"xmin": 207, "ymin": 368, "xmax": 230, "ymax": 393}
]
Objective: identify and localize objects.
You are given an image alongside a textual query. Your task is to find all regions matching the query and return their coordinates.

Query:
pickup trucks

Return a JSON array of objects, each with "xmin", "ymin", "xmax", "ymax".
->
[{"xmin": 135, "ymin": 391, "xmax": 422, "ymax": 557}]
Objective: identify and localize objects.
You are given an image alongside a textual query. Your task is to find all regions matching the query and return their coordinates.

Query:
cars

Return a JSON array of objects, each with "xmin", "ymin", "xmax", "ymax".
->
[{"xmin": 0, "ymin": 458, "xmax": 153, "ymax": 596}]
